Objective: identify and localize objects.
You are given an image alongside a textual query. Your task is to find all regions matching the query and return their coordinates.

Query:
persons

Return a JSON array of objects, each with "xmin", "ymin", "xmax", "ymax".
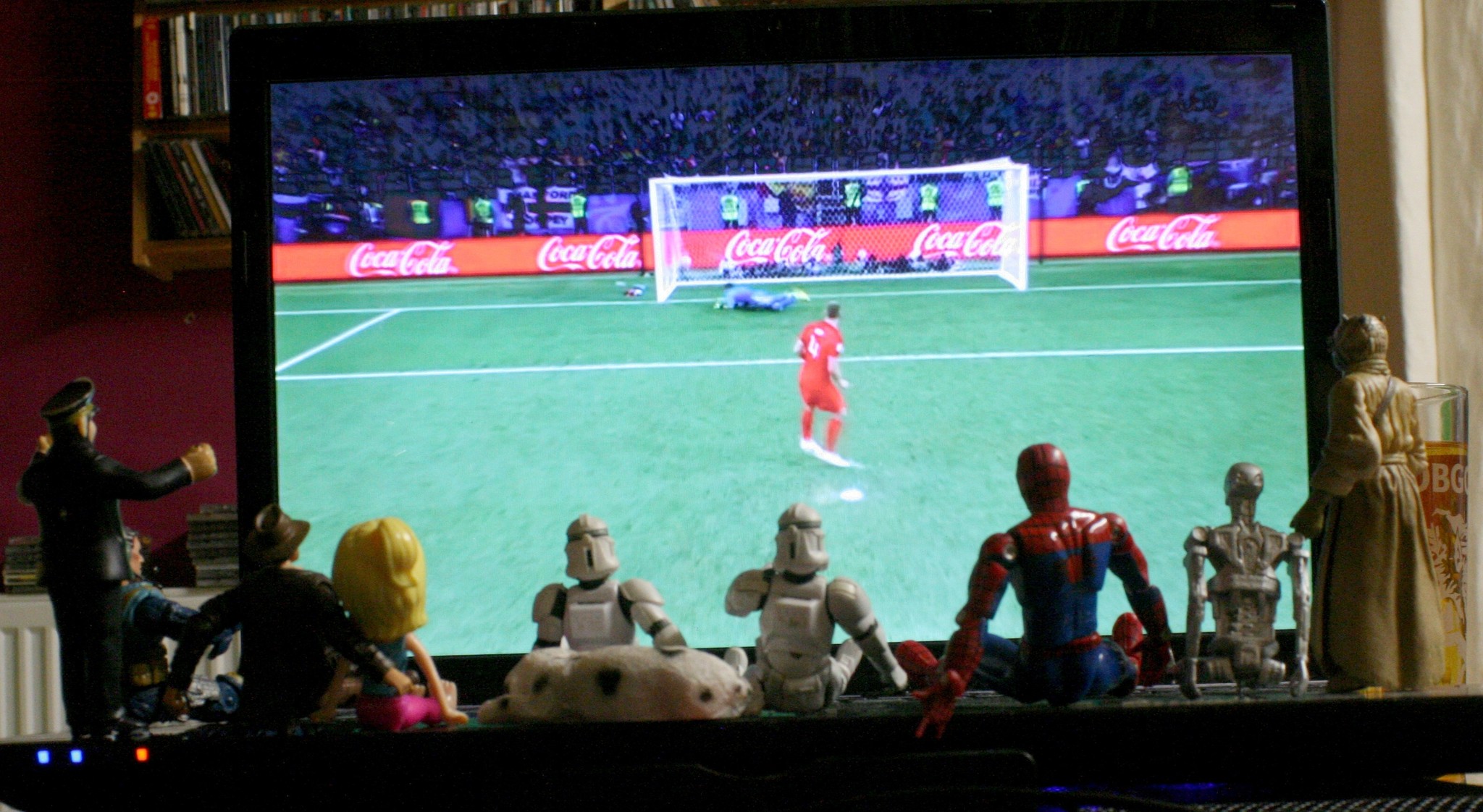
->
[
  {"xmin": 794, "ymin": 306, "xmax": 851, "ymax": 468},
  {"xmin": 272, "ymin": 51, "xmax": 1300, "ymax": 310},
  {"xmin": 528, "ymin": 444, "xmax": 1312, "ymax": 745},
  {"xmin": 16, "ymin": 378, "xmax": 470, "ymax": 735},
  {"xmin": 1289, "ymin": 314, "xmax": 1451, "ymax": 695}
]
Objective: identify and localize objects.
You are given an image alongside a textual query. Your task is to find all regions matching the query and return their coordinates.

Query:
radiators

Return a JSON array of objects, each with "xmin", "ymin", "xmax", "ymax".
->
[{"xmin": 0, "ymin": 588, "xmax": 242, "ymax": 741}]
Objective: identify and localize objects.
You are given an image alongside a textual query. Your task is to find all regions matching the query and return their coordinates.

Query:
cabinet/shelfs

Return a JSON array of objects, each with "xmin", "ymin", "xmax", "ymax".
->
[{"xmin": 131, "ymin": 0, "xmax": 784, "ymax": 282}]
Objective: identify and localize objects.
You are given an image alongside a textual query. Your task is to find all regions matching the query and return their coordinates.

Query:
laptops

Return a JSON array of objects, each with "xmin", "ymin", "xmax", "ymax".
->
[{"xmin": 0, "ymin": 1, "xmax": 1479, "ymax": 812}]
